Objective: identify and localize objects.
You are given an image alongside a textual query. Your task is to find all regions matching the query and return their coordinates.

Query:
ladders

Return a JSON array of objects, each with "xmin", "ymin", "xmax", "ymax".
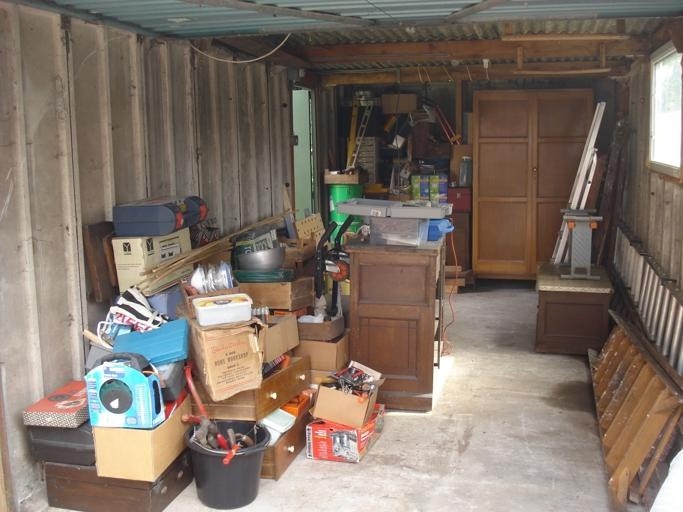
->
[
  {"xmin": 346, "ymin": 101, "xmax": 373, "ymax": 175},
  {"xmin": 607, "ymin": 218, "xmax": 682, "ymax": 377},
  {"xmin": 586, "ymin": 308, "xmax": 682, "ymax": 506},
  {"xmin": 550, "ymin": 99, "xmax": 607, "ymax": 264}
]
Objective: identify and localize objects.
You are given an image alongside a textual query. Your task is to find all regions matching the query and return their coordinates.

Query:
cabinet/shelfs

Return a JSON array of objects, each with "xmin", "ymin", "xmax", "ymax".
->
[
  {"xmin": 533, "ymin": 261, "xmax": 614, "ymax": 356},
  {"xmin": 344, "ymin": 234, "xmax": 446, "ymax": 412},
  {"xmin": 472, "ymin": 87, "xmax": 593, "ymax": 280}
]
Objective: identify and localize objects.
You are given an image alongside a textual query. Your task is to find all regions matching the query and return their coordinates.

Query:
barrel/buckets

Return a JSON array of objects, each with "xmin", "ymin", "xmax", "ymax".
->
[{"xmin": 184, "ymin": 420, "xmax": 271, "ymax": 509}]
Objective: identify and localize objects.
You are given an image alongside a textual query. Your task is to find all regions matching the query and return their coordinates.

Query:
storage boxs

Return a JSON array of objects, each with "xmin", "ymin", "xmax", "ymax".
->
[
  {"xmin": 324, "ymin": 168, "xmax": 369, "ymax": 184},
  {"xmin": 154, "ymin": 359, "xmax": 186, "ymax": 402},
  {"xmin": 305, "ymin": 402, "xmax": 383, "ymax": 464},
  {"xmin": 296, "ymin": 316, "xmax": 344, "ymax": 341},
  {"xmin": 189, "ymin": 319, "xmax": 267, "ymax": 403},
  {"xmin": 447, "ymin": 187, "xmax": 471, "ymax": 213},
  {"xmin": 178, "ymin": 277, "xmax": 240, "ymax": 319},
  {"xmin": 21, "ymin": 381, "xmax": 89, "ymax": 429},
  {"xmin": 82, "ymin": 360, "xmax": 165, "ymax": 429},
  {"xmin": 111, "ymin": 226, "xmax": 194, "ymax": 297},
  {"xmin": 312, "ymin": 378, "xmax": 385, "ymax": 430},
  {"xmin": 262, "ymin": 315, "xmax": 299, "ymax": 380},
  {"xmin": 27, "ymin": 419, "xmax": 95, "ymax": 466},
  {"xmin": 310, "ymin": 370, "xmax": 337, "ymax": 385},
  {"xmin": 44, "ymin": 447, "xmax": 194, "ymax": 512},
  {"xmin": 335, "ymin": 197, "xmax": 452, "ymax": 219},
  {"xmin": 92, "ymin": 393, "xmax": 194, "ymax": 482},
  {"xmin": 238, "ymin": 276, "xmax": 314, "ymax": 312},
  {"xmin": 112, "ymin": 318, "xmax": 190, "ymax": 366},
  {"xmin": 293, "ymin": 327, "xmax": 350, "ymax": 371},
  {"xmin": 191, "ymin": 354, "xmax": 311, "ymax": 424},
  {"xmin": 380, "ymin": 93, "xmax": 417, "ymax": 113},
  {"xmin": 260, "ymin": 401, "xmax": 314, "ymax": 482}
]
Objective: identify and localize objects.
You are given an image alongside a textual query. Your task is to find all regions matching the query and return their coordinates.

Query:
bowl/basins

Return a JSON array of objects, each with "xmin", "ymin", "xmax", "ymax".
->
[{"xmin": 237, "ymin": 246, "xmax": 286, "ymax": 272}]
